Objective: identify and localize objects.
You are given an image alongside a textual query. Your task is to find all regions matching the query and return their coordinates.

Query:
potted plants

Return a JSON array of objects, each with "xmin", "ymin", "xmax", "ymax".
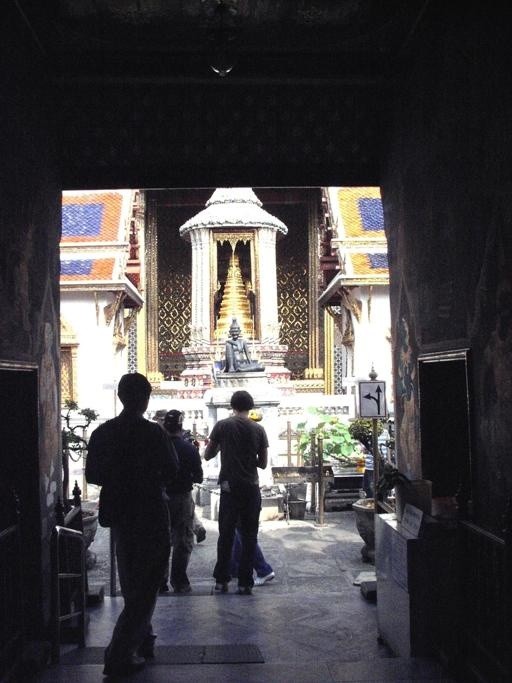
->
[
  {"xmin": 347, "ymin": 417, "xmax": 395, "ymax": 563},
  {"xmin": 60, "ymin": 398, "xmax": 99, "ymax": 572}
]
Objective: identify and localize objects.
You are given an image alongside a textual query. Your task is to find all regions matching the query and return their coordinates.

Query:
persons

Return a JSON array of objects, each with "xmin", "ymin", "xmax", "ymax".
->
[
  {"xmin": 222, "ymin": 318, "xmax": 265, "ymax": 372},
  {"xmin": 150, "ymin": 408, "xmax": 207, "ymax": 544},
  {"xmin": 162, "ymin": 410, "xmax": 205, "ymax": 596},
  {"xmin": 201, "ymin": 390, "xmax": 271, "ymax": 596},
  {"xmin": 84, "ymin": 371, "xmax": 180, "ymax": 676},
  {"xmin": 229, "ymin": 528, "xmax": 276, "ymax": 587}
]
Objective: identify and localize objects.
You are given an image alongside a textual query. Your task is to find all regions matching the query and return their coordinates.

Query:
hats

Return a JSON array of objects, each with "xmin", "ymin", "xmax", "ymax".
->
[{"xmin": 152, "ymin": 409, "xmax": 182, "ymax": 425}]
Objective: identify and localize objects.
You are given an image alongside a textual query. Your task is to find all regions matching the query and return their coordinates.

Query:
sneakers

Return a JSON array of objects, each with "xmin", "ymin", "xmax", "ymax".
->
[
  {"xmin": 253, "ymin": 572, "xmax": 275, "ymax": 585},
  {"xmin": 236, "ymin": 586, "xmax": 252, "ymax": 594},
  {"xmin": 130, "ymin": 647, "xmax": 154, "ymax": 667},
  {"xmin": 213, "ymin": 582, "xmax": 228, "ymax": 592},
  {"xmin": 197, "ymin": 527, "xmax": 206, "ymax": 542}
]
